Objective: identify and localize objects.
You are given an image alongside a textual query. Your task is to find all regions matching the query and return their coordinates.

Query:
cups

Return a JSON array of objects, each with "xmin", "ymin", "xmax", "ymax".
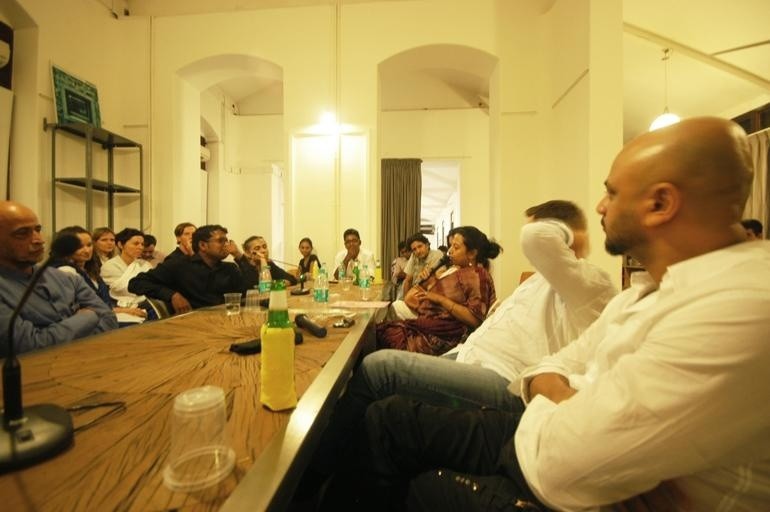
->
[
  {"xmin": 223, "ymin": 291, "xmax": 243, "ymax": 318},
  {"xmin": 163, "ymin": 385, "xmax": 236, "ymax": 492},
  {"xmin": 244, "ymin": 288, "xmax": 261, "ymax": 314}
]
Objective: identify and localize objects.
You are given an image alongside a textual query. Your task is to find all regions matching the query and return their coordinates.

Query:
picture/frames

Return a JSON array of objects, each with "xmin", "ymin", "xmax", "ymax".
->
[{"xmin": 48, "ymin": 60, "xmax": 104, "ymax": 128}]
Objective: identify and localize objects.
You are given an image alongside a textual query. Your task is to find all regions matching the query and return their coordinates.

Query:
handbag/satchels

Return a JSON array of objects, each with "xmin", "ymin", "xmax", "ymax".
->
[{"xmin": 405, "ymin": 283, "xmax": 426, "ymax": 310}]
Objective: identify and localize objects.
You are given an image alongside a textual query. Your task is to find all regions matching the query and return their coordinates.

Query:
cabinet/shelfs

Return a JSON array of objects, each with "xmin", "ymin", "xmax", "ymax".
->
[{"xmin": 45, "ymin": 122, "xmax": 143, "ymax": 262}]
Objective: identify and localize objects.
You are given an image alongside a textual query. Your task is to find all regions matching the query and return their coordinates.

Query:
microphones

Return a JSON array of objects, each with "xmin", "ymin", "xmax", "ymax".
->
[
  {"xmin": 417, "ymin": 253, "xmax": 451, "ymax": 285},
  {"xmin": 241, "ymin": 298, "xmax": 270, "ymax": 307},
  {"xmin": 252, "ymin": 250, "xmax": 310, "ymax": 295},
  {"xmin": 295, "ymin": 313, "xmax": 327, "ymax": 338},
  {"xmin": 0, "ymin": 231, "xmax": 81, "ymax": 473}
]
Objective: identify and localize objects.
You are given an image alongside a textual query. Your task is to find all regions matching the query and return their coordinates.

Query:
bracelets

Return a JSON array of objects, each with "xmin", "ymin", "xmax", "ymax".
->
[{"xmin": 451, "ymin": 303, "xmax": 456, "ymax": 313}]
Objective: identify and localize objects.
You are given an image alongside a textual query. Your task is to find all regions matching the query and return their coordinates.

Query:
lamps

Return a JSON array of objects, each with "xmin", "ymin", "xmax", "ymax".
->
[{"xmin": 649, "ymin": 41, "xmax": 682, "ymax": 133}]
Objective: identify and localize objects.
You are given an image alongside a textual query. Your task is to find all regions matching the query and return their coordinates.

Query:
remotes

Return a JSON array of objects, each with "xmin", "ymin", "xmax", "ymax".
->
[{"xmin": 229, "ymin": 328, "xmax": 303, "ymax": 356}]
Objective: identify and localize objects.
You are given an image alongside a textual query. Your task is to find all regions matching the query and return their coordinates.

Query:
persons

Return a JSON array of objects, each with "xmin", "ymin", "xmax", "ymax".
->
[
  {"xmin": 127, "ymin": 222, "xmax": 296, "ymax": 316},
  {"xmin": 3, "ymin": 199, "xmax": 164, "ymax": 352},
  {"xmin": 333, "ymin": 229, "xmax": 375, "ymax": 280},
  {"xmin": 295, "ymin": 237, "xmax": 321, "ymax": 280},
  {"xmin": 316, "ymin": 116, "xmax": 769, "ymax": 511},
  {"xmin": 378, "ymin": 226, "xmax": 504, "ymax": 356},
  {"xmin": 343, "ymin": 201, "xmax": 610, "ymax": 426},
  {"xmin": 384, "ymin": 228, "xmax": 459, "ymax": 321},
  {"xmin": 742, "ymin": 219, "xmax": 763, "ymax": 240}
]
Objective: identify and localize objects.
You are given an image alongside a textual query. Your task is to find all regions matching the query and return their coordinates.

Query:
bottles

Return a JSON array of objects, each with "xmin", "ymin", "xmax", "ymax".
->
[
  {"xmin": 312, "ymin": 267, "xmax": 330, "ymax": 323},
  {"xmin": 339, "ymin": 260, "xmax": 373, "ymax": 302},
  {"xmin": 257, "ymin": 278, "xmax": 299, "ymax": 412},
  {"xmin": 258, "ymin": 264, "xmax": 272, "ymax": 294}
]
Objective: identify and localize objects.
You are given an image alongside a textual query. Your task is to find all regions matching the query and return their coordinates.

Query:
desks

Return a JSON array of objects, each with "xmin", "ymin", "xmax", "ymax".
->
[{"xmin": 1, "ymin": 279, "xmax": 397, "ymax": 512}]
